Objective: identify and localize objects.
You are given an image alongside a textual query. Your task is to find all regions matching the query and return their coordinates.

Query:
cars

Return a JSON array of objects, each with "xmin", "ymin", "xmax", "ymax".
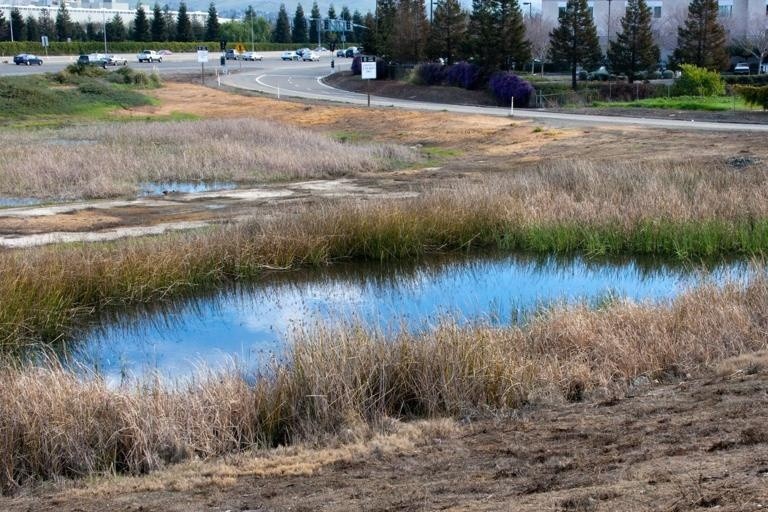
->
[
  {"xmin": 225, "ymin": 48, "xmax": 262, "ymax": 61},
  {"xmin": 137, "ymin": 49, "xmax": 172, "ymax": 63},
  {"xmin": 14, "ymin": 54, "xmax": 43, "ymax": 66},
  {"xmin": 734, "ymin": 63, "xmax": 768, "ymax": 75},
  {"xmin": 337, "ymin": 46, "xmax": 385, "ymax": 75},
  {"xmin": 281, "ymin": 47, "xmax": 326, "ymax": 62},
  {"xmin": 78, "ymin": 53, "xmax": 127, "ymax": 67},
  {"xmin": 575, "ymin": 65, "xmax": 673, "ymax": 82}
]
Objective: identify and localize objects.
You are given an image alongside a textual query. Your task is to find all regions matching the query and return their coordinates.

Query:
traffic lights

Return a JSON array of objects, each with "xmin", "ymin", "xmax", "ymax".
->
[{"xmin": 220, "ymin": 40, "xmax": 227, "ymax": 50}]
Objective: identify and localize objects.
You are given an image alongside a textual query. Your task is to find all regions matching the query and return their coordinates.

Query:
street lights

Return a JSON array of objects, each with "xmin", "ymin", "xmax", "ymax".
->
[
  {"xmin": 430, "ymin": 0, "xmax": 438, "ymax": 23},
  {"xmin": 523, "ymin": 2, "xmax": 532, "ymax": 19}
]
